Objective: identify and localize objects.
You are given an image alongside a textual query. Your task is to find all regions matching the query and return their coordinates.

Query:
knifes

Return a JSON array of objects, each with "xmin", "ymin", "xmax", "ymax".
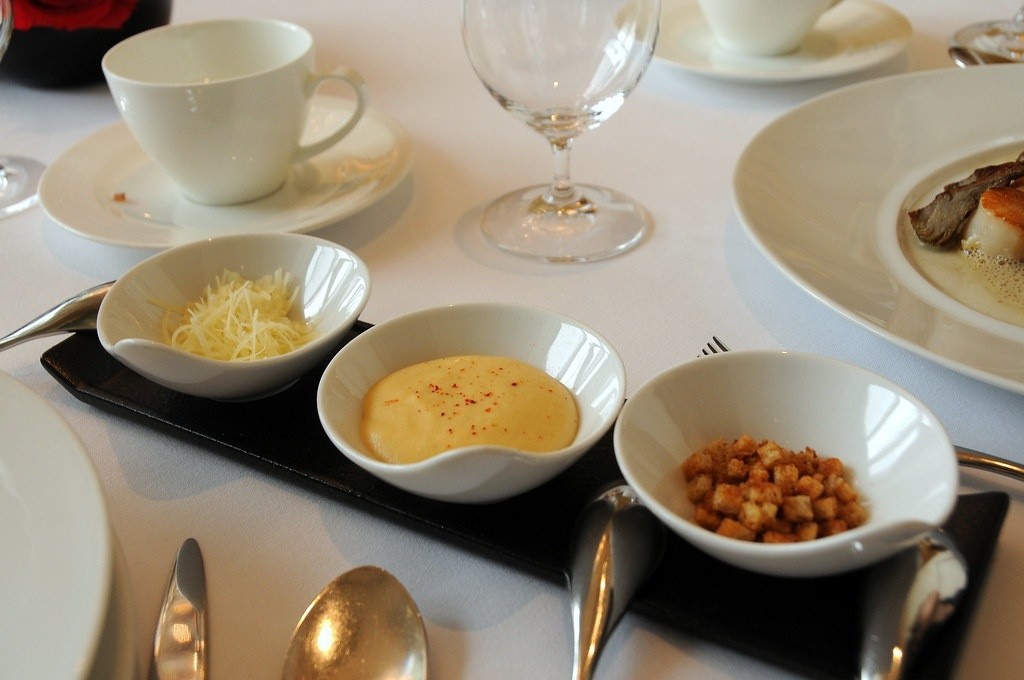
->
[{"xmin": 147, "ymin": 537, "xmax": 208, "ymax": 680}]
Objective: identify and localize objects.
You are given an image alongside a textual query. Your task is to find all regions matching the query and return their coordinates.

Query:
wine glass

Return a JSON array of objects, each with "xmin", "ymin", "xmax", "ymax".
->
[
  {"xmin": 461, "ymin": 0, "xmax": 661, "ymax": 264},
  {"xmin": 0, "ymin": 0, "xmax": 48, "ymax": 219}
]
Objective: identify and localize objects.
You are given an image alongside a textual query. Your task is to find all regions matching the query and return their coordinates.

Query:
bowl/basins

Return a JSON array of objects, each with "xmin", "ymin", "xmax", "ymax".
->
[
  {"xmin": 614, "ymin": 349, "xmax": 960, "ymax": 578},
  {"xmin": 316, "ymin": 300, "xmax": 625, "ymax": 505},
  {"xmin": 96, "ymin": 232, "xmax": 372, "ymax": 403}
]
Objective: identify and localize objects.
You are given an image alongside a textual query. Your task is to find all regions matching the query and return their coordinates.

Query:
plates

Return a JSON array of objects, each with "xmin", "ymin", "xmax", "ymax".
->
[
  {"xmin": 728, "ymin": 61, "xmax": 1024, "ymax": 394},
  {"xmin": 615, "ymin": 0, "xmax": 914, "ymax": 83},
  {"xmin": 0, "ymin": 370, "xmax": 114, "ymax": 680},
  {"xmin": 36, "ymin": 93, "xmax": 415, "ymax": 248}
]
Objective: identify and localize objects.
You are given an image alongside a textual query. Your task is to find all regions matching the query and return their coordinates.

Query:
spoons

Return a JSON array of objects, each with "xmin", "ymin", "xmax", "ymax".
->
[
  {"xmin": 858, "ymin": 528, "xmax": 968, "ymax": 680},
  {"xmin": 568, "ymin": 482, "xmax": 657, "ymax": 680},
  {"xmin": 280, "ymin": 566, "xmax": 429, "ymax": 680},
  {"xmin": 949, "ymin": 46, "xmax": 984, "ymax": 68}
]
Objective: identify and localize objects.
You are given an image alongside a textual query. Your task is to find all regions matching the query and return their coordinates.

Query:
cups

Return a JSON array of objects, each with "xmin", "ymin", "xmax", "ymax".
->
[
  {"xmin": 101, "ymin": 16, "xmax": 369, "ymax": 206},
  {"xmin": 699, "ymin": 0, "xmax": 840, "ymax": 57}
]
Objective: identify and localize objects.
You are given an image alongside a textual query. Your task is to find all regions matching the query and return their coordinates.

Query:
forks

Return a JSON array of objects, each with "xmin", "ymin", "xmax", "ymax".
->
[{"xmin": 694, "ymin": 335, "xmax": 1024, "ymax": 480}]
